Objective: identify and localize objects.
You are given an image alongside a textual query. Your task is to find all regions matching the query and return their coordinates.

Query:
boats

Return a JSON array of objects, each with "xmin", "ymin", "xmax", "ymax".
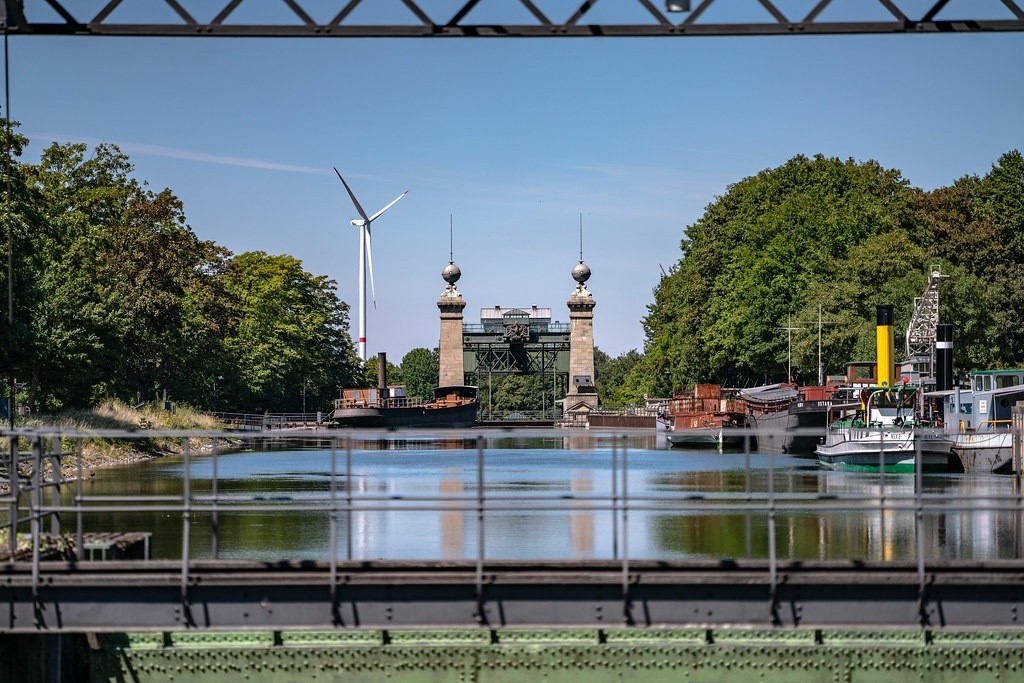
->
[
  {"xmin": 332, "ymin": 352, "xmax": 479, "ymax": 429},
  {"xmin": 812, "ymin": 378, "xmax": 953, "ymax": 470},
  {"xmin": 920, "ymin": 367, "xmax": 1024, "ymax": 472}
]
